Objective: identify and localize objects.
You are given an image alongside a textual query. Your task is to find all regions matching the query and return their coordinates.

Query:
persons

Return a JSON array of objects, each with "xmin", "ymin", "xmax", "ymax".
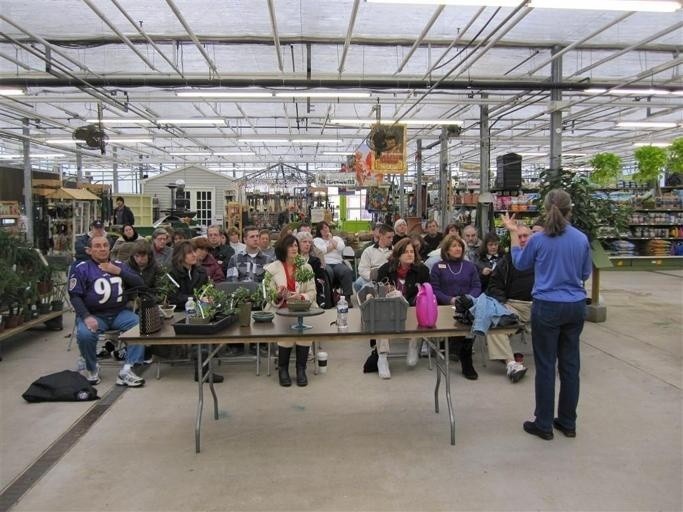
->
[
  {"xmin": 207, "ymin": 222, "xmax": 354, "ymax": 310},
  {"xmin": 167, "ymin": 224, "xmax": 236, "ymax": 382},
  {"xmin": 113, "ymin": 196, "xmax": 134, "ymax": 225},
  {"xmin": 262, "ymin": 234, "xmax": 318, "ymax": 389},
  {"xmin": 68, "ymin": 236, "xmax": 146, "ymax": 387},
  {"xmin": 354, "ymin": 219, "xmax": 545, "ymax": 294},
  {"xmin": 487, "ymin": 223, "xmax": 535, "ymax": 384},
  {"xmin": 501, "ymin": 189, "xmax": 593, "ymax": 440},
  {"xmin": 68, "ymin": 220, "xmax": 174, "ymax": 361},
  {"xmin": 430, "ymin": 233, "xmax": 482, "ymax": 379},
  {"xmin": 372, "ymin": 237, "xmax": 430, "ymax": 378}
]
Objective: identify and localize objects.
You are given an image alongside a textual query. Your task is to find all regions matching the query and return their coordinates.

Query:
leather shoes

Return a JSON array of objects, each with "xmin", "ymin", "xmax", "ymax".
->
[
  {"xmin": 195, "ymin": 369, "xmax": 224, "ymax": 383},
  {"xmin": 524, "ymin": 421, "xmax": 553, "ymax": 440},
  {"xmin": 553, "ymin": 418, "xmax": 576, "ymax": 437}
]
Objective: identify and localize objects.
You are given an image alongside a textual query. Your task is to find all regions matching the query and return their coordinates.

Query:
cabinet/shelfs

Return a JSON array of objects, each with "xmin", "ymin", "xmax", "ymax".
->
[
  {"xmin": 49, "ymin": 200, "xmax": 97, "ymax": 255},
  {"xmin": 493, "ymin": 191, "xmax": 682, "ymax": 259},
  {"xmin": 113, "ymin": 194, "xmax": 154, "ymax": 226}
]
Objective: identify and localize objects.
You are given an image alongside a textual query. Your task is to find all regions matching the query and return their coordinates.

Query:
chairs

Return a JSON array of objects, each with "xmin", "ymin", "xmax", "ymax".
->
[
  {"xmin": 263, "ymin": 278, "xmax": 321, "ymax": 375},
  {"xmin": 376, "ymin": 337, "xmax": 434, "ymax": 370},
  {"xmin": 354, "ymin": 249, "xmax": 365, "ymax": 280},
  {"xmin": 358, "ymin": 234, "xmax": 372, "ymax": 246},
  {"xmin": 202, "ymin": 282, "xmax": 261, "ymax": 376},
  {"xmin": 67, "ymin": 264, "xmax": 77, "ymax": 353}
]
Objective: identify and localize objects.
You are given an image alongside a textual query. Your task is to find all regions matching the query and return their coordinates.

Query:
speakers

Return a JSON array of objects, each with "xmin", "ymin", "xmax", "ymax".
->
[{"xmin": 496, "ymin": 153, "xmax": 522, "ymax": 188}]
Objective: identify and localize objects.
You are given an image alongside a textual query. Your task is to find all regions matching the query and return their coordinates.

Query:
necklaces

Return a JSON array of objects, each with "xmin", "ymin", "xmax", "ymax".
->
[{"xmin": 447, "ymin": 261, "xmax": 463, "ymax": 275}]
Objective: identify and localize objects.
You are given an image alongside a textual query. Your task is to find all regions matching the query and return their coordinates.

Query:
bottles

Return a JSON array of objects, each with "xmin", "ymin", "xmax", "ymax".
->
[
  {"xmin": 183, "ymin": 296, "xmax": 197, "ymax": 325},
  {"xmin": 335, "ymin": 295, "xmax": 349, "ymax": 329}
]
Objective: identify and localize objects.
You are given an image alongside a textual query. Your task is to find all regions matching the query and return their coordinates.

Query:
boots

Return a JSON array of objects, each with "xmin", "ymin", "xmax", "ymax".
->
[
  {"xmin": 459, "ymin": 337, "xmax": 478, "ymax": 380},
  {"xmin": 296, "ymin": 345, "xmax": 310, "ymax": 386},
  {"xmin": 279, "ymin": 346, "xmax": 293, "ymax": 385}
]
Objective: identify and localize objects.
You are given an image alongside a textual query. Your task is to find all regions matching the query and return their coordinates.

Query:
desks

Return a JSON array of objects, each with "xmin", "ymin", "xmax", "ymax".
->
[{"xmin": 118, "ymin": 304, "xmax": 525, "ymax": 454}]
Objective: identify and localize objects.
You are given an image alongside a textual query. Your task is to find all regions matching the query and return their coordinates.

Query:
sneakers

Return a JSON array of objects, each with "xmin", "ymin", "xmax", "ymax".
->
[
  {"xmin": 87, "ymin": 362, "xmax": 101, "ymax": 385},
  {"xmin": 116, "ymin": 369, "xmax": 145, "ymax": 386},
  {"xmin": 105, "ymin": 340, "xmax": 152, "ymax": 364},
  {"xmin": 507, "ymin": 362, "xmax": 527, "ymax": 382},
  {"xmin": 406, "ymin": 346, "xmax": 419, "ymax": 366},
  {"xmin": 377, "ymin": 359, "xmax": 390, "ymax": 379}
]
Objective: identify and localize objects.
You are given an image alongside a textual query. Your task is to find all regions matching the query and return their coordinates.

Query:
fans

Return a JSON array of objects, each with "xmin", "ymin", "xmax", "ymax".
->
[
  {"xmin": 365, "ymin": 125, "xmax": 400, "ymax": 152},
  {"xmin": 71, "ymin": 125, "xmax": 108, "ymax": 151}
]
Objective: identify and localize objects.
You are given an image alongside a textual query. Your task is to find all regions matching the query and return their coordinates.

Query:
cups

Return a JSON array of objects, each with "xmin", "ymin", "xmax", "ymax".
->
[{"xmin": 316, "ymin": 351, "xmax": 330, "ymax": 374}]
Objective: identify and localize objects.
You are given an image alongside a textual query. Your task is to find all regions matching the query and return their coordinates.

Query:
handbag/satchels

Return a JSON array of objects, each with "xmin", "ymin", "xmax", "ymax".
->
[
  {"xmin": 140, "ymin": 298, "xmax": 161, "ymax": 334},
  {"xmin": 23, "ymin": 370, "xmax": 101, "ymax": 402},
  {"xmin": 416, "ymin": 283, "xmax": 438, "ymax": 327}
]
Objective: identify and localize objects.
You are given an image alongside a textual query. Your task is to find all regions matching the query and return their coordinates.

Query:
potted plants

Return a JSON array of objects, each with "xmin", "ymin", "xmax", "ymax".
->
[
  {"xmin": 0, "ymin": 229, "xmax": 63, "ymax": 333},
  {"xmin": 190, "ymin": 270, "xmax": 279, "ymax": 327},
  {"xmin": 287, "ymin": 255, "xmax": 315, "ymax": 310},
  {"xmin": 152, "ymin": 264, "xmax": 180, "ymax": 318}
]
